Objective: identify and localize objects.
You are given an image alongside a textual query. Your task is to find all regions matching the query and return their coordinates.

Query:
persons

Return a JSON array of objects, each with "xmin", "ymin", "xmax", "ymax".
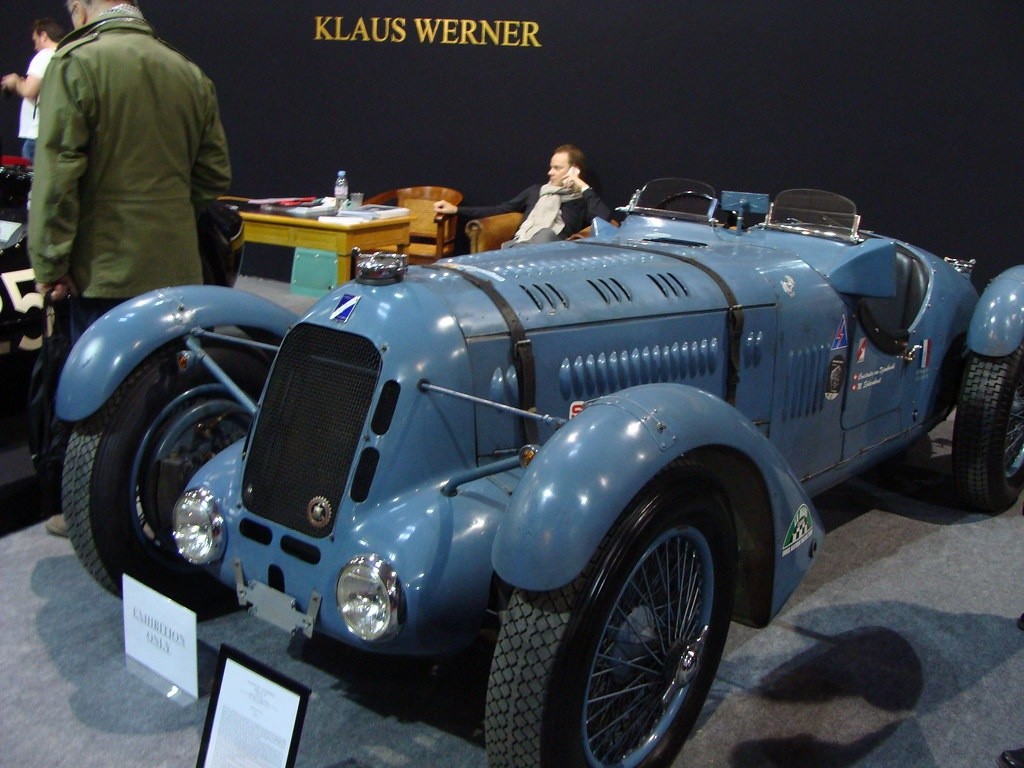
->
[
  {"xmin": 2, "ymin": 17, "xmax": 68, "ymax": 163},
  {"xmin": 432, "ymin": 144, "xmax": 612, "ymax": 251},
  {"xmin": 27, "ymin": 0, "xmax": 232, "ymax": 538}
]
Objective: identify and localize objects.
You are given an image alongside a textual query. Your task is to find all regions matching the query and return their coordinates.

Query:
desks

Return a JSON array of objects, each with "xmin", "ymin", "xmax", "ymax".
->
[{"xmin": 218, "ymin": 196, "xmax": 417, "ymax": 291}]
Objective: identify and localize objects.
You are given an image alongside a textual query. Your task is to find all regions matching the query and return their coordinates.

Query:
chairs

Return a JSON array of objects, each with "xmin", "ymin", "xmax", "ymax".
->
[
  {"xmin": 466, "ymin": 211, "xmax": 620, "ymax": 253},
  {"xmin": 354, "ymin": 186, "xmax": 464, "ymax": 278},
  {"xmin": 629, "ymin": 178, "xmax": 720, "ymax": 229},
  {"xmin": 764, "ymin": 188, "xmax": 862, "ymax": 244}
]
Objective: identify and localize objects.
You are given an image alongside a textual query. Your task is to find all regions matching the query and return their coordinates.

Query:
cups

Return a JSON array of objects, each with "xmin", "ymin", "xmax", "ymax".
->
[{"xmin": 350, "ymin": 192, "xmax": 364, "ymax": 208}]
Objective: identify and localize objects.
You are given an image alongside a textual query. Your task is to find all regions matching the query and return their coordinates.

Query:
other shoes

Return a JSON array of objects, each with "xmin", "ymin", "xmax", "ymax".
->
[{"xmin": 45, "ymin": 512, "xmax": 70, "ymax": 537}]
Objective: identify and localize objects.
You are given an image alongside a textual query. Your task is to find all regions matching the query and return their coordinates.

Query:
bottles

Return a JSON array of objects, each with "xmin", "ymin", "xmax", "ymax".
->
[{"xmin": 333, "ymin": 170, "xmax": 348, "ymax": 210}]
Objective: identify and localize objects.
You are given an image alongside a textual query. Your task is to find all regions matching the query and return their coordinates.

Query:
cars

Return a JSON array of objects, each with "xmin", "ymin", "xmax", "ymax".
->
[
  {"xmin": 1, "ymin": 163, "xmax": 245, "ymax": 417},
  {"xmin": 53, "ymin": 177, "xmax": 1024, "ymax": 768}
]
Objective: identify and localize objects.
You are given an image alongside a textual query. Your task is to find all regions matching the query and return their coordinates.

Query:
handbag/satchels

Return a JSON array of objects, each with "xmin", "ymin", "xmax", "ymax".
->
[
  {"xmin": 21, "ymin": 284, "xmax": 73, "ymax": 474},
  {"xmin": 198, "ymin": 198, "xmax": 246, "ymax": 285}
]
